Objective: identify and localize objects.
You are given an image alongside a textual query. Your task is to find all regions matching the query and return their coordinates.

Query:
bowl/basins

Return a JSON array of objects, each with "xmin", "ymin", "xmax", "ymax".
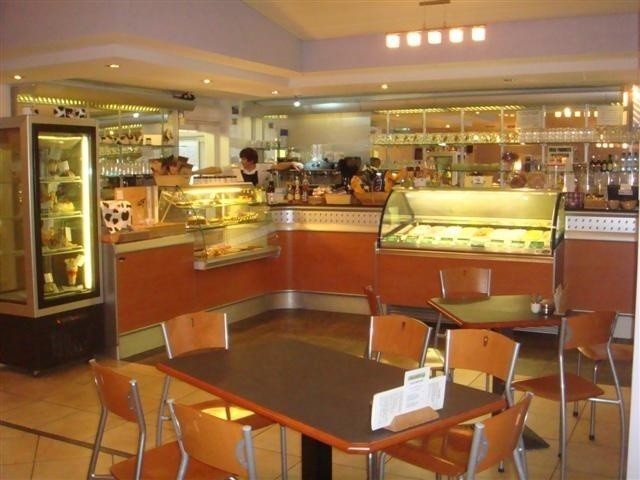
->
[
  {"xmin": 621, "ymin": 200, "xmax": 638, "ymax": 210},
  {"xmin": 606, "ymin": 200, "xmax": 620, "ymax": 210}
]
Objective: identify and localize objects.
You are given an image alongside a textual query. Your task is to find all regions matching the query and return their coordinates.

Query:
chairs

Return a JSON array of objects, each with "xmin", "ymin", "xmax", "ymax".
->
[
  {"xmin": 465, "ymin": 387, "xmax": 531, "ymax": 479},
  {"xmin": 87, "ymin": 360, "xmax": 232, "ymax": 479},
  {"xmin": 433, "ymin": 267, "xmax": 495, "ymax": 392},
  {"xmin": 361, "ymin": 282, "xmax": 445, "ymax": 378},
  {"xmin": 165, "ymin": 395, "xmax": 256, "ymax": 479},
  {"xmin": 496, "ymin": 308, "xmax": 617, "ymax": 480},
  {"xmin": 377, "ymin": 325, "xmax": 519, "ymax": 480},
  {"xmin": 364, "ymin": 315, "xmax": 430, "ymax": 369},
  {"xmin": 573, "ymin": 340, "xmax": 630, "ymax": 438},
  {"xmin": 154, "ymin": 313, "xmax": 289, "ymax": 479}
]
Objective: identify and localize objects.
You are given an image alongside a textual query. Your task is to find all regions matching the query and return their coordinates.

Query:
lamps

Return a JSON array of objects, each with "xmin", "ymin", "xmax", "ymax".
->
[{"xmin": 385, "ymin": 0, "xmax": 487, "ymax": 49}]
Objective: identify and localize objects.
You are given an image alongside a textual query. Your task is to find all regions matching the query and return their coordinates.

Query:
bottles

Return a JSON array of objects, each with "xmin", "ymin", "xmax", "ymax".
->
[
  {"xmin": 287, "ymin": 174, "xmax": 308, "ymax": 203},
  {"xmin": 267, "ymin": 174, "xmax": 276, "ymax": 206},
  {"xmin": 590, "ymin": 152, "xmax": 638, "ymax": 172}
]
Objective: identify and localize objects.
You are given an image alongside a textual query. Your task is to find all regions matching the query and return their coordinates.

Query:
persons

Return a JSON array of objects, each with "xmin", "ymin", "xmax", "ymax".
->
[{"xmin": 191, "ymin": 147, "xmax": 304, "ymax": 190}]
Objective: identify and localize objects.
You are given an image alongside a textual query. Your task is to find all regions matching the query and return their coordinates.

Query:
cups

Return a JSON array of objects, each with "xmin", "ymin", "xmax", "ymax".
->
[{"xmin": 531, "ymin": 302, "xmax": 540, "ymax": 313}]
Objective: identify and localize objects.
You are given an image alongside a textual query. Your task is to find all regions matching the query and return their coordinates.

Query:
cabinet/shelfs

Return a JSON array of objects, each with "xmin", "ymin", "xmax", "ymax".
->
[
  {"xmin": 157, "ymin": 183, "xmax": 282, "ymax": 271},
  {"xmin": 376, "ymin": 186, "xmax": 566, "ymax": 257},
  {"xmin": 10, "ymin": 79, "xmax": 195, "ymax": 189}
]
